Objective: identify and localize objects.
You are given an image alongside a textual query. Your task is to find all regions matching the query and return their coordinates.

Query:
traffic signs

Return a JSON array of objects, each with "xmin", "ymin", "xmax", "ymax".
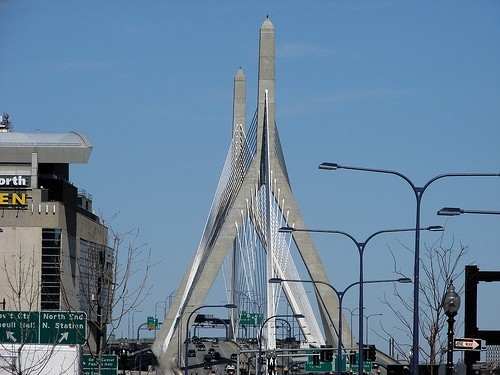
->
[
  {"xmin": 81, "ymin": 367, "xmax": 118, "ymax": 375},
  {"xmin": 39, "ymin": 310, "xmax": 89, "ymax": 345},
  {"xmin": 454, "ymin": 338, "xmax": 483, "ymax": 352},
  {"xmin": 82, "ymin": 354, "xmax": 118, "ymax": 369},
  {"xmin": 0, "ymin": 310, "xmax": 39, "ymax": 346}
]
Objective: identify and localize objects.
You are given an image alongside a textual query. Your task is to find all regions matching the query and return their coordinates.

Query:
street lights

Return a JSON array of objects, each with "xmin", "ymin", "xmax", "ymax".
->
[
  {"xmin": 267, "ymin": 278, "xmax": 414, "ymax": 375},
  {"xmin": 441, "ymin": 277, "xmax": 463, "ymax": 375},
  {"xmin": 277, "ymin": 225, "xmax": 446, "ymax": 375},
  {"xmin": 337, "ymin": 305, "xmax": 366, "ymax": 351},
  {"xmin": 185, "ymin": 303, "xmax": 239, "ymax": 375},
  {"xmin": 266, "ymin": 348, "xmax": 278, "ymax": 375},
  {"xmin": 317, "ymin": 161, "xmax": 500, "ymax": 375},
  {"xmin": 132, "ymin": 309, "xmax": 144, "ymax": 341},
  {"xmin": 258, "ymin": 313, "xmax": 305, "ymax": 375},
  {"xmin": 351, "ymin": 313, "xmax": 383, "ymax": 345}
]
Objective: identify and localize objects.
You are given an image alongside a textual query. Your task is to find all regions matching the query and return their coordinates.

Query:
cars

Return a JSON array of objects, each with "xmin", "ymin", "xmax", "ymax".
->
[{"xmin": 185, "ymin": 335, "xmax": 238, "ymax": 375}]
